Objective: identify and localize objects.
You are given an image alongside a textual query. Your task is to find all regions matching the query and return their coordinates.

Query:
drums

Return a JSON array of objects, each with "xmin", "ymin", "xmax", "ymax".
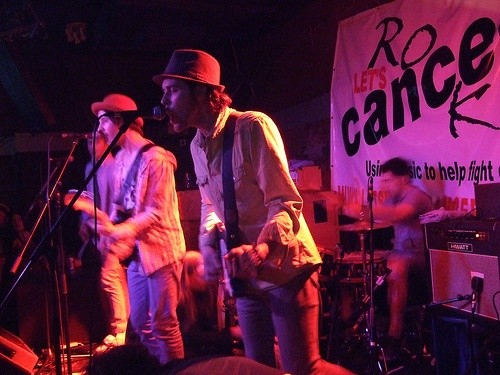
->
[
  {"xmin": 336, "ymin": 254, "xmax": 384, "ymax": 283},
  {"xmin": 317, "ymin": 246, "xmax": 336, "ymax": 281}
]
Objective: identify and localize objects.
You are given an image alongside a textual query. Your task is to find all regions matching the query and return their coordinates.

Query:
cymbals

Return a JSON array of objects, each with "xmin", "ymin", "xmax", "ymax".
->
[{"xmin": 339, "ymin": 219, "xmax": 392, "ymax": 231}]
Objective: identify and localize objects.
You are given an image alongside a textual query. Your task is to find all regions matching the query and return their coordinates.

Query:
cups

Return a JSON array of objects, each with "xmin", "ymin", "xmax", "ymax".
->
[{"xmin": 181, "ymin": 170, "xmax": 194, "ymax": 189}]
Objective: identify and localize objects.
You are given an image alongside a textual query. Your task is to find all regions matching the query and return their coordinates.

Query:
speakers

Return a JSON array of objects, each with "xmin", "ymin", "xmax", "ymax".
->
[
  {"xmin": 0, "ymin": 327, "xmax": 39, "ymax": 375},
  {"xmin": 428, "ymin": 250, "xmax": 500, "ymax": 324}
]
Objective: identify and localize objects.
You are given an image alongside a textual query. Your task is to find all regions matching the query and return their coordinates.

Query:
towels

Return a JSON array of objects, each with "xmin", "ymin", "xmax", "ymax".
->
[{"xmin": 419, "ymin": 207, "xmax": 467, "ymax": 224}]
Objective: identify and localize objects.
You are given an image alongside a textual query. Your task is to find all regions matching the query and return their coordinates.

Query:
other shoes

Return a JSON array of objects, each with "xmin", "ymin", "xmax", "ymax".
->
[
  {"xmin": 379, "ymin": 335, "xmax": 401, "ymax": 361},
  {"xmin": 321, "ymin": 315, "xmax": 357, "ymax": 339},
  {"xmin": 95, "ymin": 331, "xmax": 126, "ymax": 352}
]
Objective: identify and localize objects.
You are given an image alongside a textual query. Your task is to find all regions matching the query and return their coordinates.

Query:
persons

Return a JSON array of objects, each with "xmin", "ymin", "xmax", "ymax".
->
[
  {"xmin": 85, "ymin": 94, "xmax": 188, "ymax": 368},
  {"xmin": 74, "ymin": 128, "xmax": 130, "ymax": 356},
  {"xmin": 158, "ymin": 48, "xmax": 356, "ymax": 374},
  {"xmin": 340, "ymin": 157, "xmax": 436, "ymax": 350}
]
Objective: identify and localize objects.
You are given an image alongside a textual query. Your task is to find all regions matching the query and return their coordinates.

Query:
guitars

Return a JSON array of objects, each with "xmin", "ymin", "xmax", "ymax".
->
[
  {"xmin": 64, "ymin": 189, "xmax": 137, "ymax": 266},
  {"xmin": 214, "ymin": 221, "xmax": 261, "ymax": 298}
]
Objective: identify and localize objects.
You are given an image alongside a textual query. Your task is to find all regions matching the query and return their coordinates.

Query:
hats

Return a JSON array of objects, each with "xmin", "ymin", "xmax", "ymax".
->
[
  {"xmin": 91, "ymin": 94, "xmax": 144, "ymax": 127},
  {"xmin": 152, "ymin": 50, "xmax": 225, "ymax": 93}
]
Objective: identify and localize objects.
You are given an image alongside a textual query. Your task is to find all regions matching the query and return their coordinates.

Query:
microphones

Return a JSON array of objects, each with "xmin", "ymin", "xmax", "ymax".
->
[
  {"xmin": 50, "ymin": 156, "xmax": 74, "ymax": 162},
  {"xmin": 55, "ymin": 131, "xmax": 100, "ymax": 139},
  {"xmin": 367, "ymin": 177, "xmax": 373, "ymax": 201},
  {"xmin": 103, "ymin": 105, "xmax": 167, "ymax": 121},
  {"xmin": 471, "ymin": 276, "xmax": 482, "ymax": 313},
  {"xmin": 337, "ymin": 242, "xmax": 351, "ymax": 255}
]
